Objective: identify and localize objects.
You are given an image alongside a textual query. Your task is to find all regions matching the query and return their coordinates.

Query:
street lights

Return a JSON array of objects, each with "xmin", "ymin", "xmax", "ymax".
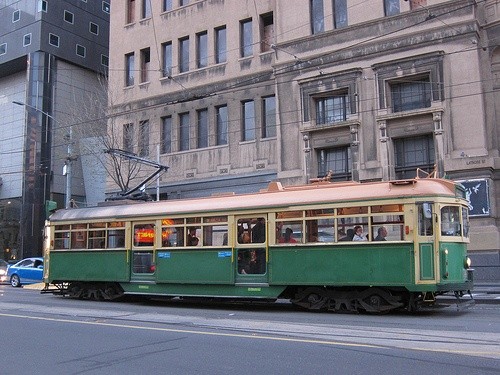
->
[{"xmin": 12, "ymin": 101, "xmax": 71, "ymax": 137}]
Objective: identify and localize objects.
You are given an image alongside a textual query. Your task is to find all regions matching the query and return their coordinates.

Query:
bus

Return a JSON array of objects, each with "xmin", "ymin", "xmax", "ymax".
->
[{"xmin": 41, "ymin": 178, "xmax": 473, "ymax": 313}]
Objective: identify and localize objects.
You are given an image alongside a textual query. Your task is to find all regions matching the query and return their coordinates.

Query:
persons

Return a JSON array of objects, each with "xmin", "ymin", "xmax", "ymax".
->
[
  {"xmin": 428, "ymin": 216, "xmax": 444, "ymax": 235},
  {"xmin": 238, "ymin": 218, "xmax": 266, "ymax": 274},
  {"xmin": 279, "ymin": 228, "xmax": 297, "ymax": 243},
  {"xmin": 373, "ymin": 226, "xmax": 388, "ymax": 240},
  {"xmin": 337, "ymin": 229, "xmax": 350, "ymax": 241},
  {"xmin": 352, "ymin": 225, "xmax": 368, "ymax": 241},
  {"xmin": 190, "ymin": 237, "xmax": 199, "ymax": 245},
  {"xmin": 187, "ymin": 227, "xmax": 196, "ymax": 245}
]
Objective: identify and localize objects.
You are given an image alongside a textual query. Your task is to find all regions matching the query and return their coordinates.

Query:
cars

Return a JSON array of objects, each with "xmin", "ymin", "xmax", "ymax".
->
[
  {"xmin": 5, "ymin": 257, "xmax": 44, "ymax": 287},
  {"xmin": 0, "ymin": 259, "xmax": 12, "ymax": 285}
]
